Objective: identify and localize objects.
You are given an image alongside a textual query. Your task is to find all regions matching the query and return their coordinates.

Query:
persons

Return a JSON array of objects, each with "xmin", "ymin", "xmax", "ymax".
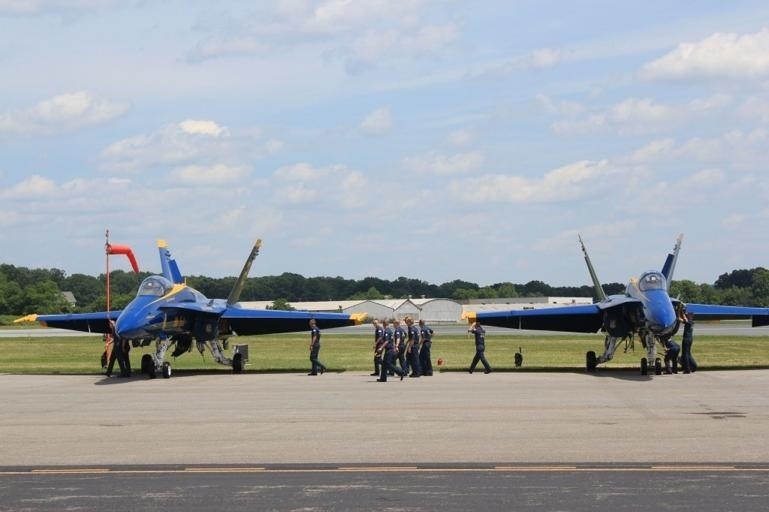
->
[
  {"xmin": 370, "ymin": 316, "xmax": 435, "ymax": 382},
  {"xmin": 307, "ymin": 319, "xmax": 326, "ymax": 375},
  {"xmin": 105, "ymin": 320, "xmax": 132, "ymax": 378},
  {"xmin": 664, "ymin": 311, "xmax": 697, "ymax": 374},
  {"xmin": 468, "ymin": 322, "xmax": 493, "ymax": 374}
]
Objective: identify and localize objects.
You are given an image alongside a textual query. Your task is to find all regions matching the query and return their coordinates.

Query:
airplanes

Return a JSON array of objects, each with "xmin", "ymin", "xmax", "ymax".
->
[
  {"xmin": 14, "ymin": 239, "xmax": 368, "ymax": 379},
  {"xmin": 462, "ymin": 232, "xmax": 769, "ymax": 375}
]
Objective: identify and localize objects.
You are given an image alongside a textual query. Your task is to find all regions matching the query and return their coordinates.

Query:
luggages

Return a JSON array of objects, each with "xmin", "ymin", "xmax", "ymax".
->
[{"xmin": 514, "ymin": 353, "xmax": 523, "ymax": 367}]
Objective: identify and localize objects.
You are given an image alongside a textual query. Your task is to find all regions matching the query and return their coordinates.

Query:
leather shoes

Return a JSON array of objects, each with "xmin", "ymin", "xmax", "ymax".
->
[
  {"xmin": 321, "ymin": 367, "xmax": 325, "ymax": 374},
  {"xmin": 467, "ymin": 369, "xmax": 473, "ymax": 373},
  {"xmin": 370, "ymin": 370, "xmax": 432, "ymax": 382},
  {"xmin": 484, "ymin": 369, "xmax": 491, "ymax": 373},
  {"xmin": 308, "ymin": 373, "xmax": 317, "ymax": 375}
]
